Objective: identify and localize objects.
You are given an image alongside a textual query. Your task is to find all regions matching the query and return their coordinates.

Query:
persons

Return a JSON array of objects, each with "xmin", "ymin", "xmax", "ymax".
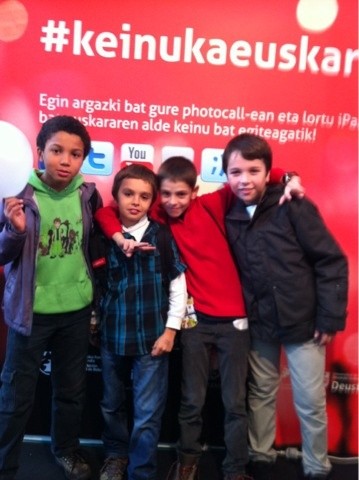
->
[
  {"xmin": 0, "ymin": 116, "xmax": 103, "ymax": 480},
  {"xmin": 89, "ymin": 163, "xmax": 189, "ymax": 480},
  {"xmin": 96, "ymin": 157, "xmax": 306, "ymax": 480},
  {"xmin": 222, "ymin": 133, "xmax": 349, "ymax": 480}
]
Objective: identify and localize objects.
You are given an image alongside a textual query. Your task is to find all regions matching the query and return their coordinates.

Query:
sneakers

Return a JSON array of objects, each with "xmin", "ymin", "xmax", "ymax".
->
[
  {"xmin": 174, "ymin": 462, "xmax": 197, "ymax": 480},
  {"xmin": 55, "ymin": 453, "xmax": 90, "ymax": 479},
  {"xmin": 100, "ymin": 456, "xmax": 127, "ymax": 480},
  {"xmin": 225, "ymin": 472, "xmax": 246, "ymax": 480}
]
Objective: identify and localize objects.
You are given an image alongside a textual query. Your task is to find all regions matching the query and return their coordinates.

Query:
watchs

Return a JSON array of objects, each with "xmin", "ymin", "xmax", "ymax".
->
[{"xmin": 282, "ymin": 170, "xmax": 298, "ymax": 182}]
